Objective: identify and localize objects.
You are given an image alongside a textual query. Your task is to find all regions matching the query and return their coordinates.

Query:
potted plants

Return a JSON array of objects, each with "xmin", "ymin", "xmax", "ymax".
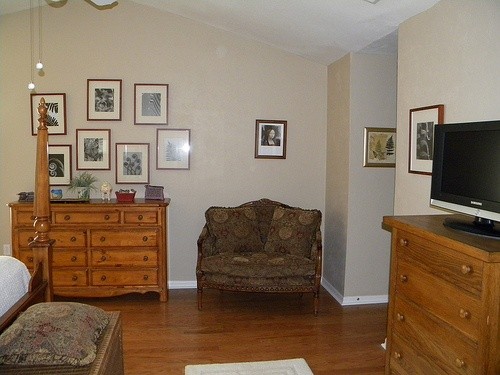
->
[{"xmin": 66, "ymin": 172, "xmax": 98, "ymax": 201}]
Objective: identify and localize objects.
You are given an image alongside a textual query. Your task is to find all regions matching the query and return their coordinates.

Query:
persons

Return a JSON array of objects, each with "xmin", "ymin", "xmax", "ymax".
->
[{"xmin": 263, "ymin": 128, "xmax": 278, "ymax": 146}]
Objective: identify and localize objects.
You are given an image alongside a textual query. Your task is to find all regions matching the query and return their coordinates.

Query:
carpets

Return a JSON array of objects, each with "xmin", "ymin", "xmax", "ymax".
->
[{"xmin": 184, "ymin": 358, "xmax": 314, "ymax": 375}]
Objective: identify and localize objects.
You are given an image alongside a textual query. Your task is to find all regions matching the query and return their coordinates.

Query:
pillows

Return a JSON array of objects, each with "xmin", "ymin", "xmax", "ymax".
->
[
  {"xmin": 0, "ymin": 301, "xmax": 109, "ymax": 365},
  {"xmin": 205, "ymin": 206, "xmax": 263, "ymax": 253},
  {"xmin": 264, "ymin": 205, "xmax": 322, "ymax": 258}
]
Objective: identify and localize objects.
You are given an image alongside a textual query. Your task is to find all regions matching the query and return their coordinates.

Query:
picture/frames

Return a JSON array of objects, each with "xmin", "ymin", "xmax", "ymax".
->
[
  {"xmin": 30, "ymin": 93, "xmax": 67, "ymax": 136},
  {"xmin": 115, "ymin": 142, "xmax": 150, "ymax": 184},
  {"xmin": 86, "ymin": 78, "xmax": 122, "ymax": 121},
  {"xmin": 134, "ymin": 83, "xmax": 169, "ymax": 125},
  {"xmin": 156, "ymin": 128, "xmax": 191, "ymax": 170},
  {"xmin": 363, "ymin": 127, "xmax": 396, "ymax": 168},
  {"xmin": 408, "ymin": 104, "xmax": 444, "ymax": 175},
  {"xmin": 255, "ymin": 120, "xmax": 287, "ymax": 159},
  {"xmin": 76, "ymin": 129, "xmax": 111, "ymax": 170},
  {"xmin": 48, "ymin": 144, "xmax": 72, "ymax": 186}
]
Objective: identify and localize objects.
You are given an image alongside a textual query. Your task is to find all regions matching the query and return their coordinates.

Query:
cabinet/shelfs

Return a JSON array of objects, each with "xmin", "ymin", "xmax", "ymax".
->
[
  {"xmin": 383, "ymin": 213, "xmax": 500, "ymax": 375},
  {"xmin": 8, "ymin": 198, "xmax": 170, "ymax": 302}
]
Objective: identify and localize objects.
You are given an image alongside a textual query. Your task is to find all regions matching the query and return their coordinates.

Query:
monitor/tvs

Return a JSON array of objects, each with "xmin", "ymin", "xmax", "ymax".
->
[{"xmin": 430, "ymin": 119, "xmax": 500, "ymax": 239}]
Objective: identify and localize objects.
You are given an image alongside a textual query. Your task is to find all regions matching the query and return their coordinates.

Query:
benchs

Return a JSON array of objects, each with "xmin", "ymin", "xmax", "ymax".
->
[
  {"xmin": 196, "ymin": 198, "xmax": 322, "ymax": 317},
  {"xmin": 0, "ymin": 310, "xmax": 124, "ymax": 375}
]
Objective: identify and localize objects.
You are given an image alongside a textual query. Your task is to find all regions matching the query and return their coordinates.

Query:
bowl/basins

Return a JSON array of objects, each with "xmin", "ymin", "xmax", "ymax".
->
[{"xmin": 115, "ymin": 191, "xmax": 136, "ymax": 201}]
